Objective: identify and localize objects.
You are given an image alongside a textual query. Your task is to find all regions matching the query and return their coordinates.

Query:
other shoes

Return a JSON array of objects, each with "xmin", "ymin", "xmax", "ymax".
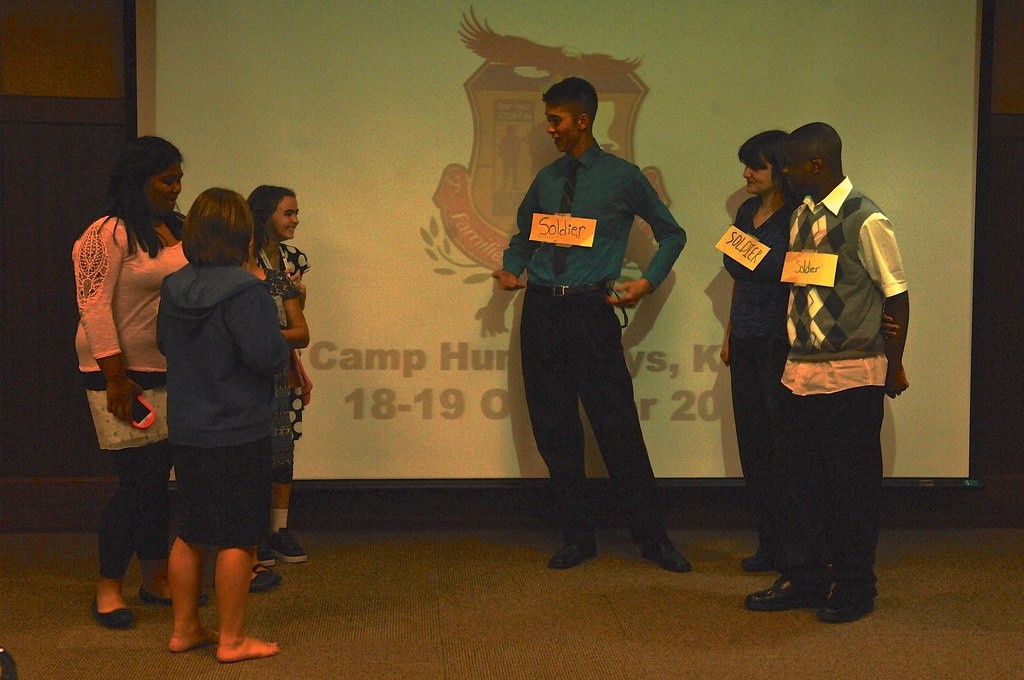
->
[
  {"xmin": 251, "ymin": 563, "xmax": 282, "ymax": 586},
  {"xmin": 264, "ymin": 526, "xmax": 307, "ymax": 563},
  {"xmin": 249, "ymin": 573, "xmax": 274, "ymax": 592},
  {"xmin": 91, "ymin": 595, "xmax": 136, "ymax": 629},
  {"xmin": 256, "ymin": 534, "xmax": 276, "ymax": 566},
  {"xmin": 137, "ymin": 585, "xmax": 209, "ymax": 608}
]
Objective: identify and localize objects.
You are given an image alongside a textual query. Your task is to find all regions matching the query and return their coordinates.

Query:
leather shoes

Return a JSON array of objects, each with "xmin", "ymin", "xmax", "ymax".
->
[
  {"xmin": 816, "ymin": 582, "xmax": 877, "ymax": 625},
  {"xmin": 639, "ymin": 539, "xmax": 692, "ymax": 572},
  {"xmin": 744, "ymin": 576, "xmax": 827, "ymax": 611},
  {"xmin": 741, "ymin": 549, "xmax": 777, "ymax": 571},
  {"xmin": 547, "ymin": 538, "xmax": 597, "ymax": 569}
]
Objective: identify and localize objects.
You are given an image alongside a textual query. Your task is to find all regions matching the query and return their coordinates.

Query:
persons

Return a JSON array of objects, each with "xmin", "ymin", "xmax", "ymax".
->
[
  {"xmin": 242, "ymin": 186, "xmax": 310, "ymax": 567},
  {"xmin": 742, "ymin": 117, "xmax": 912, "ymax": 623},
  {"xmin": 155, "ymin": 186, "xmax": 282, "ymax": 664},
  {"xmin": 209, "ymin": 203, "xmax": 310, "ymax": 594},
  {"xmin": 493, "ymin": 76, "xmax": 693, "ymax": 573},
  {"xmin": 70, "ymin": 134, "xmax": 209, "ymax": 628},
  {"xmin": 718, "ymin": 129, "xmax": 830, "ymax": 573}
]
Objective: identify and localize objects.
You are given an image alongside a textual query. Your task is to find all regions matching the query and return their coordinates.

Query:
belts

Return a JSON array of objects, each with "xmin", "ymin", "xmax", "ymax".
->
[{"xmin": 526, "ymin": 279, "xmax": 607, "ymax": 298}]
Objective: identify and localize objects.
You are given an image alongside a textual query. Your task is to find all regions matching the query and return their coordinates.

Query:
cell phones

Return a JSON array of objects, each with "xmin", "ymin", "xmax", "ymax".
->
[{"xmin": 131, "ymin": 395, "xmax": 155, "ymax": 429}]
